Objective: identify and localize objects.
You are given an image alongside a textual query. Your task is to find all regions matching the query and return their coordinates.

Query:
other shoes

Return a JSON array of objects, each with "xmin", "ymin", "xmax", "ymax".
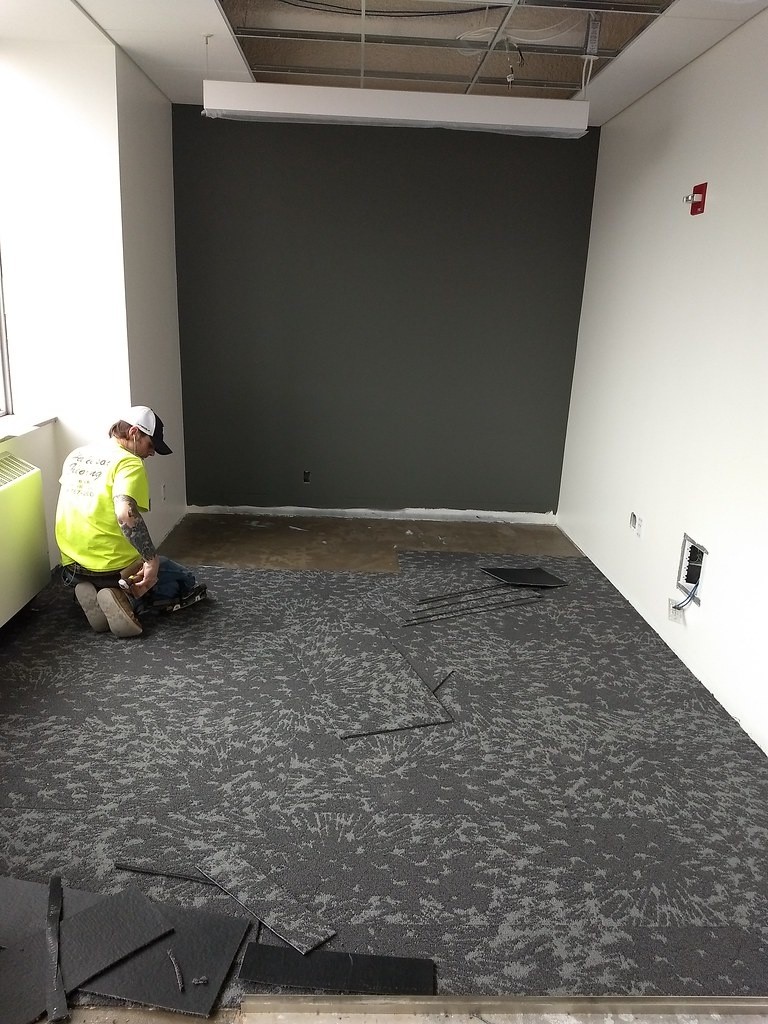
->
[
  {"xmin": 75, "ymin": 581, "xmax": 110, "ymax": 634},
  {"xmin": 96, "ymin": 586, "xmax": 144, "ymax": 637}
]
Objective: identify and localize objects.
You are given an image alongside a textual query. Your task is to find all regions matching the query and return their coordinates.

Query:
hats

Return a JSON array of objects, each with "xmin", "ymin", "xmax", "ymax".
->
[{"xmin": 122, "ymin": 405, "xmax": 173, "ymax": 456}]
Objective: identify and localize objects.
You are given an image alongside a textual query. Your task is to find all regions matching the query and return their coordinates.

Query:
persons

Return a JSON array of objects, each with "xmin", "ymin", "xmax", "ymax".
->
[{"xmin": 55, "ymin": 405, "xmax": 196, "ymax": 637}]
